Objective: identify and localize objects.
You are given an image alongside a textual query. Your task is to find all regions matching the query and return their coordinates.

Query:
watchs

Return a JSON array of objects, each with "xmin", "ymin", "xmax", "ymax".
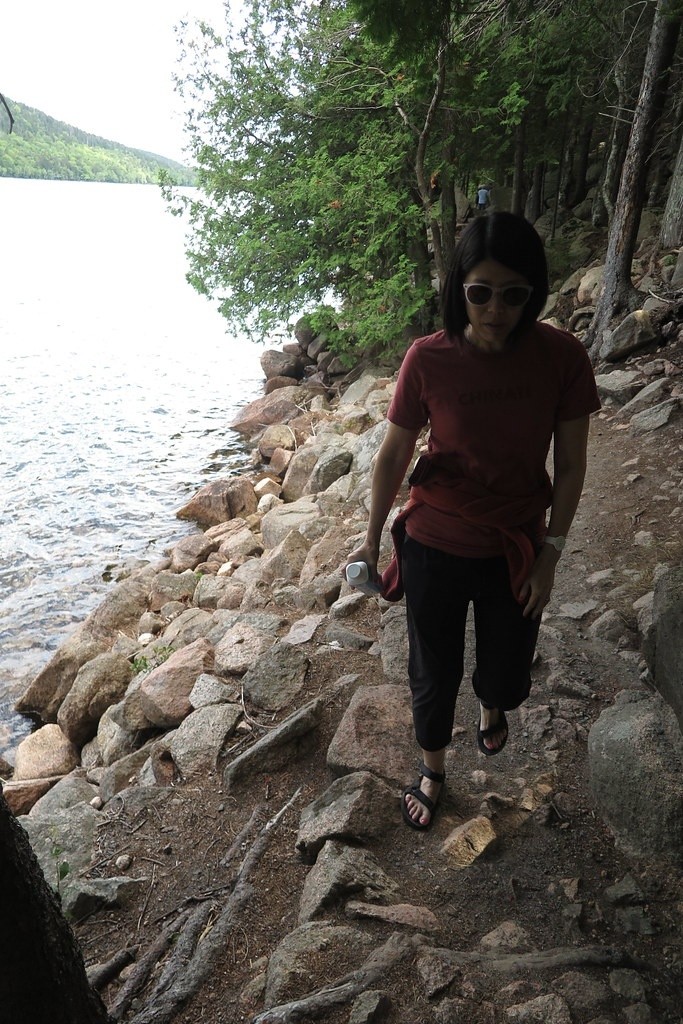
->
[{"xmin": 544, "ymin": 536, "xmax": 566, "ymax": 552}]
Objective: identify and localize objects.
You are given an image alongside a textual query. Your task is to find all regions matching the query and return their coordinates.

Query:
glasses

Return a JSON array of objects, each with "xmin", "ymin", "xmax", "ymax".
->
[{"xmin": 461, "ymin": 278, "xmax": 534, "ymax": 310}]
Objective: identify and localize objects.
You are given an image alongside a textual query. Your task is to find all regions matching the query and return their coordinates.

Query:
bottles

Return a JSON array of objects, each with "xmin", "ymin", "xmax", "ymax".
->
[{"xmin": 345, "ymin": 562, "xmax": 383, "ymax": 598}]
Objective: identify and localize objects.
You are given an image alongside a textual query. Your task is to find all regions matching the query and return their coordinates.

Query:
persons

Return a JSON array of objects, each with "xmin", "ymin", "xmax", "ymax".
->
[
  {"xmin": 475, "ymin": 184, "xmax": 489, "ymax": 210},
  {"xmin": 347, "ymin": 210, "xmax": 603, "ymax": 832}
]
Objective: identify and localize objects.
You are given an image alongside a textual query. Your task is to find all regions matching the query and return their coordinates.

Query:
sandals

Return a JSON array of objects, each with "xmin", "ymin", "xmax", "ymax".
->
[
  {"xmin": 400, "ymin": 761, "xmax": 446, "ymax": 832},
  {"xmin": 476, "ymin": 700, "xmax": 509, "ymax": 756}
]
